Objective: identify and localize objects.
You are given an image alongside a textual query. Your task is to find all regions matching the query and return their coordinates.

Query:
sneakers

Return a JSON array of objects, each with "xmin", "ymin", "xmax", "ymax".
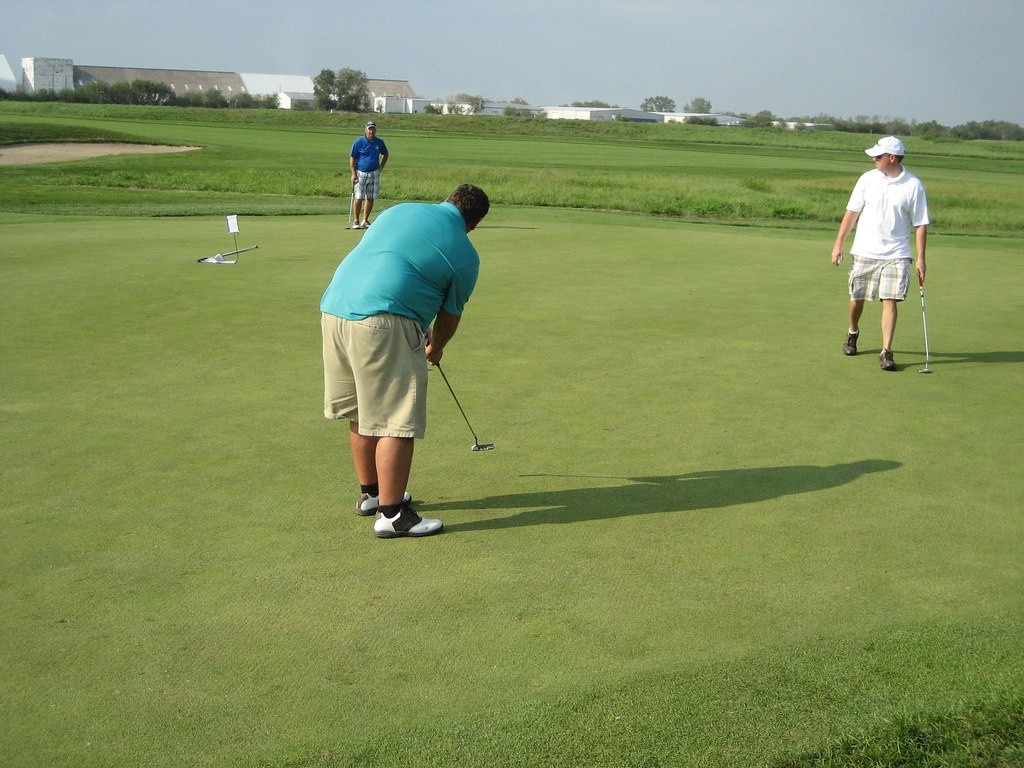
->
[
  {"xmin": 843, "ymin": 329, "xmax": 859, "ymax": 356},
  {"xmin": 373, "ymin": 498, "xmax": 443, "ymax": 538},
  {"xmin": 879, "ymin": 347, "xmax": 896, "ymax": 370},
  {"xmin": 355, "ymin": 485, "xmax": 412, "ymax": 516},
  {"xmin": 361, "ymin": 221, "xmax": 371, "ymax": 228},
  {"xmin": 352, "ymin": 221, "xmax": 360, "ymax": 229}
]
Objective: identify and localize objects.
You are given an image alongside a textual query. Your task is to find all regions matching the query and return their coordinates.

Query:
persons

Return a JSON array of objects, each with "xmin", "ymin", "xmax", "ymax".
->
[
  {"xmin": 832, "ymin": 136, "xmax": 930, "ymax": 371},
  {"xmin": 320, "ymin": 184, "xmax": 491, "ymax": 538},
  {"xmin": 350, "ymin": 121, "xmax": 389, "ymax": 229}
]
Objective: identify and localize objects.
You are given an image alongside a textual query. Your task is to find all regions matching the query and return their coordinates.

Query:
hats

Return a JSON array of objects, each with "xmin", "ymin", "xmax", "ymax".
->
[
  {"xmin": 364, "ymin": 121, "xmax": 376, "ymax": 129},
  {"xmin": 864, "ymin": 136, "xmax": 905, "ymax": 156}
]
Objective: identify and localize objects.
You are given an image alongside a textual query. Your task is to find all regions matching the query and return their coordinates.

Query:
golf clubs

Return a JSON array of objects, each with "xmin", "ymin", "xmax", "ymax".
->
[
  {"xmin": 343, "ymin": 180, "xmax": 356, "ymax": 230},
  {"xmin": 435, "ymin": 361, "xmax": 496, "ymax": 452},
  {"xmin": 917, "ymin": 269, "xmax": 934, "ymax": 374}
]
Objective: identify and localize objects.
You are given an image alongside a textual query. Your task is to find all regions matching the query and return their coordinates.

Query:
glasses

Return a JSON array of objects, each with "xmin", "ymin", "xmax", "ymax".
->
[{"xmin": 876, "ymin": 155, "xmax": 893, "ymax": 161}]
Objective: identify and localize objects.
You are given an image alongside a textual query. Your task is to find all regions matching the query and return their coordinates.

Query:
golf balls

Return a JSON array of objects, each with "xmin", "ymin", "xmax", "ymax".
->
[{"xmin": 836, "ymin": 258, "xmax": 842, "ymax": 265}]
